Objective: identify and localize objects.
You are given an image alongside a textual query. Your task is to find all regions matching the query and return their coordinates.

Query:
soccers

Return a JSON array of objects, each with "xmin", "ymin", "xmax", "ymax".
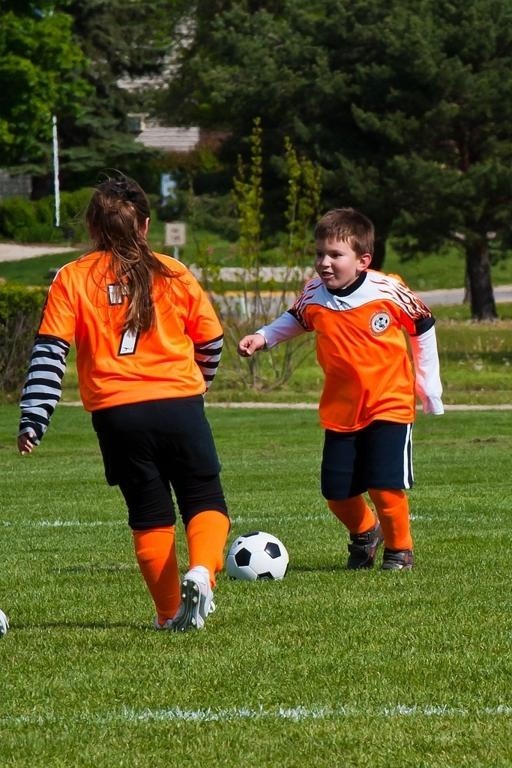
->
[{"xmin": 226, "ymin": 531, "xmax": 289, "ymax": 579}]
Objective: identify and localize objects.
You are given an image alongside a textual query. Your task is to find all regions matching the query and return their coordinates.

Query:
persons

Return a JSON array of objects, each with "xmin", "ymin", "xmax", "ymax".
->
[
  {"xmin": 15, "ymin": 166, "xmax": 233, "ymax": 636},
  {"xmin": 236, "ymin": 204, "xmax": 444, "ymax": 571}
]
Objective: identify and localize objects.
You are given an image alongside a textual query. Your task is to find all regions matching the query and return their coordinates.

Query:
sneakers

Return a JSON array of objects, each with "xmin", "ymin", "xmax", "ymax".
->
[
  {"xmin": 158, "ymin": 566, "xmax": 216, "ymax": 631},
  {"xmin": 1, "ymin": 611, "xmax": 8, "ymax": 637},
  {"xmin": 381, "ymin": 547, "xmax": 415, "ymax": 571},
  {"xmin": 347, "ymin": 510, "xmax": 384, "ymax": 570}
]
